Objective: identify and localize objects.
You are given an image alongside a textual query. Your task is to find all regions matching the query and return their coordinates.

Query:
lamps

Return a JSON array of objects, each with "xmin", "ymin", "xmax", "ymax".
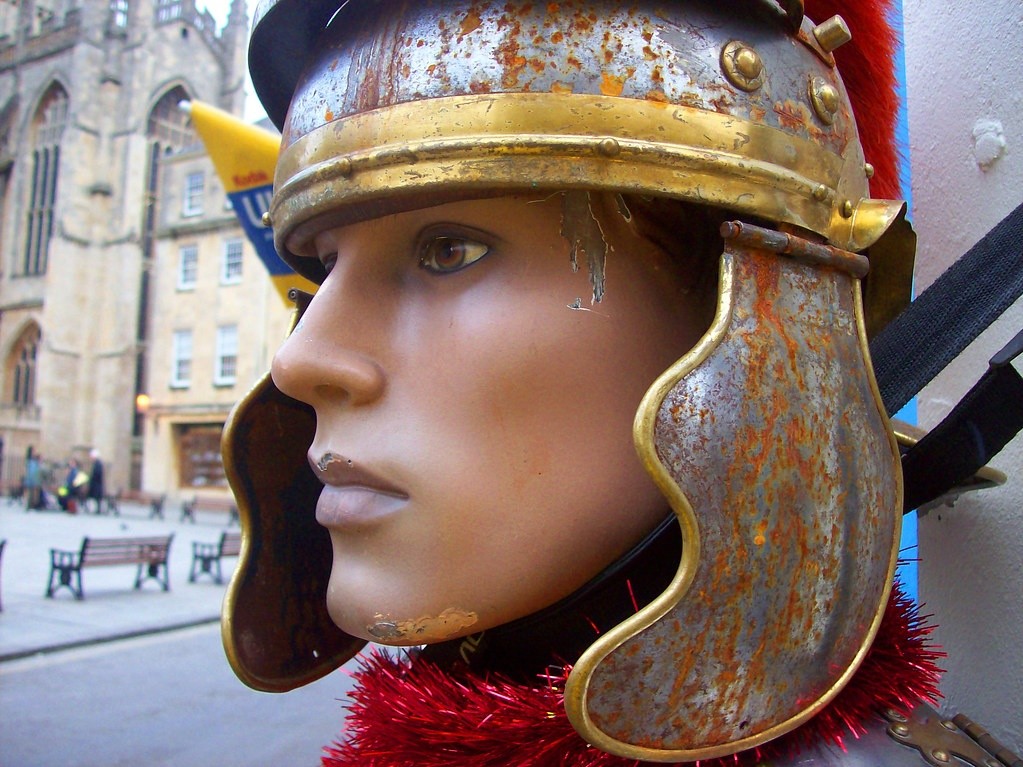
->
[{"xmin": 138, "ymin": 395, "xmax": 156, "ymax": 416}]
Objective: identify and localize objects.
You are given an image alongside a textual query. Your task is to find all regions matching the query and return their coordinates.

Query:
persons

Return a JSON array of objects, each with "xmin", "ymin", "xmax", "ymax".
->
[
  {"xmin": 24, "ymin": 446, "xmax": 104, "ymax": 515},
  {"xmin": 220, "ymin": 0, "xmax": 1023, "ymax": 767}
]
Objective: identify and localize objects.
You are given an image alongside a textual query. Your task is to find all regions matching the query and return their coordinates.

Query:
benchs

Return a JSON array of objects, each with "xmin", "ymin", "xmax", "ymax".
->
[
  {"xmin": 192, "ymin": 531, "xmax": 244, "ymax": 580},
  {"xmin": 181, "ymin": 494, "xmax": 243, "ymax": 527},
  {"xmin": 104, "ymin": 487, "xmax": 168, "ymax": 519},
  {"xmin": 46, "ymin": 531, "xmax": 176, "ymax": 600}
]
